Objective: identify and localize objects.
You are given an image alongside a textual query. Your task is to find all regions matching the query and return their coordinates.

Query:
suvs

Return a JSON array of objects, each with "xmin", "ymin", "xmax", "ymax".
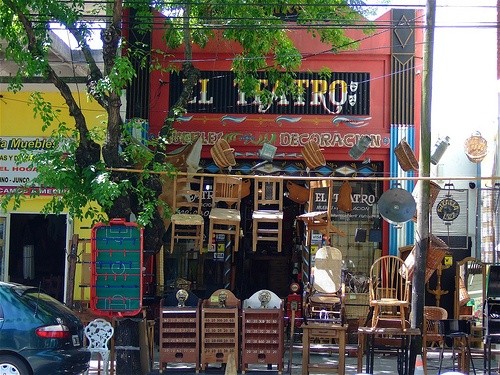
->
[{"xmin": 0, "ymin": 281, "xmax": 92, "ymax": 375}]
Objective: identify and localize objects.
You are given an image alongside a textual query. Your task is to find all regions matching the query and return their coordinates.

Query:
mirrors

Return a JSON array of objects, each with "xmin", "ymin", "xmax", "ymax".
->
[{"xmin": 454, "ymin": 257, "xmax": 486, "ymax": 348}]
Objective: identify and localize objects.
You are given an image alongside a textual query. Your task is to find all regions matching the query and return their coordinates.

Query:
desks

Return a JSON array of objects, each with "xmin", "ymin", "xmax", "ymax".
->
[
  {"xmin": 357, "ymin": 326, "xmax": 421, "ymax": 374},
  {"xmin": 298, "ymin": 322, "xmax": 349, "ymax": 375}
]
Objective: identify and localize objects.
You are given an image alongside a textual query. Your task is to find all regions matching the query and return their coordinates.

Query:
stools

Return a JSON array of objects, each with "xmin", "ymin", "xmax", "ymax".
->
[{"xmin": 438, "ymin": 319, "xmax": 476, "ymax": 375}]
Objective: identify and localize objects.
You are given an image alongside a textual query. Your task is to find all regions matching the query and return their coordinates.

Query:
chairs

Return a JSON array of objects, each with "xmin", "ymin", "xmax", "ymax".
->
[
  {"xmin": 303, "ymin": 246, "xmax": 345, "ymax": 327},
  {"xmin": 296, "ymin": 180, "xmax": 345, "ymax": 246},
  {"xmin": 170, "ymin": 176, "xmax": 204, "ymax": 254},
  {"xmin": 252, "ymin": 175, "xmax": 283, "ymax": 252},
  {"xmin": 208, "ymin": 177, "xmax": 242, "ymax": 251},
  {"xmin": 83, "ymin": 318, "xmax": 114, "ymax": 375},
  {"xmin": 422, "ymin": 306, "xmax": 448, "ymax": 359},
  {"xmin": 370, "ymin": 255, "xmax": 410, "ymax": 332}
]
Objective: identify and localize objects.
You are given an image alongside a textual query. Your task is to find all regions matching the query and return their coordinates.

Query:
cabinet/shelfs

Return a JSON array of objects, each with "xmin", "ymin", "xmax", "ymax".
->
[
  {"xmin": 160, "ymin": 288, "xmax": 200, "ymax": 373},
  {"xmin": 242, "ymin": 290, "xmax": 284, "ymax": 375},
  {"xmin": 200, "ymin": 289, "xmax": 241, "ymax": 371},
  {"xmin": 483, "ymin": 297, "xmax": 500, "ymax": 375}
]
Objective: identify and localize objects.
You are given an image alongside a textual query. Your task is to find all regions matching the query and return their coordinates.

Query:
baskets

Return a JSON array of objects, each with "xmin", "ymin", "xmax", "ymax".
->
[
  {"xmin": 394, "ymin": 138, "xmax": 420, "ymax": 171},
  {"xmin": 301, "ymin": 140, "xmax": 326, "ymax": 169},
  {"xmin": 463, "ymin": 130, "xmax": 486, "ymax": 163},
  {"xmin": 210, "ymin": 139, "xmax": 236, "ymax": 168}
]
